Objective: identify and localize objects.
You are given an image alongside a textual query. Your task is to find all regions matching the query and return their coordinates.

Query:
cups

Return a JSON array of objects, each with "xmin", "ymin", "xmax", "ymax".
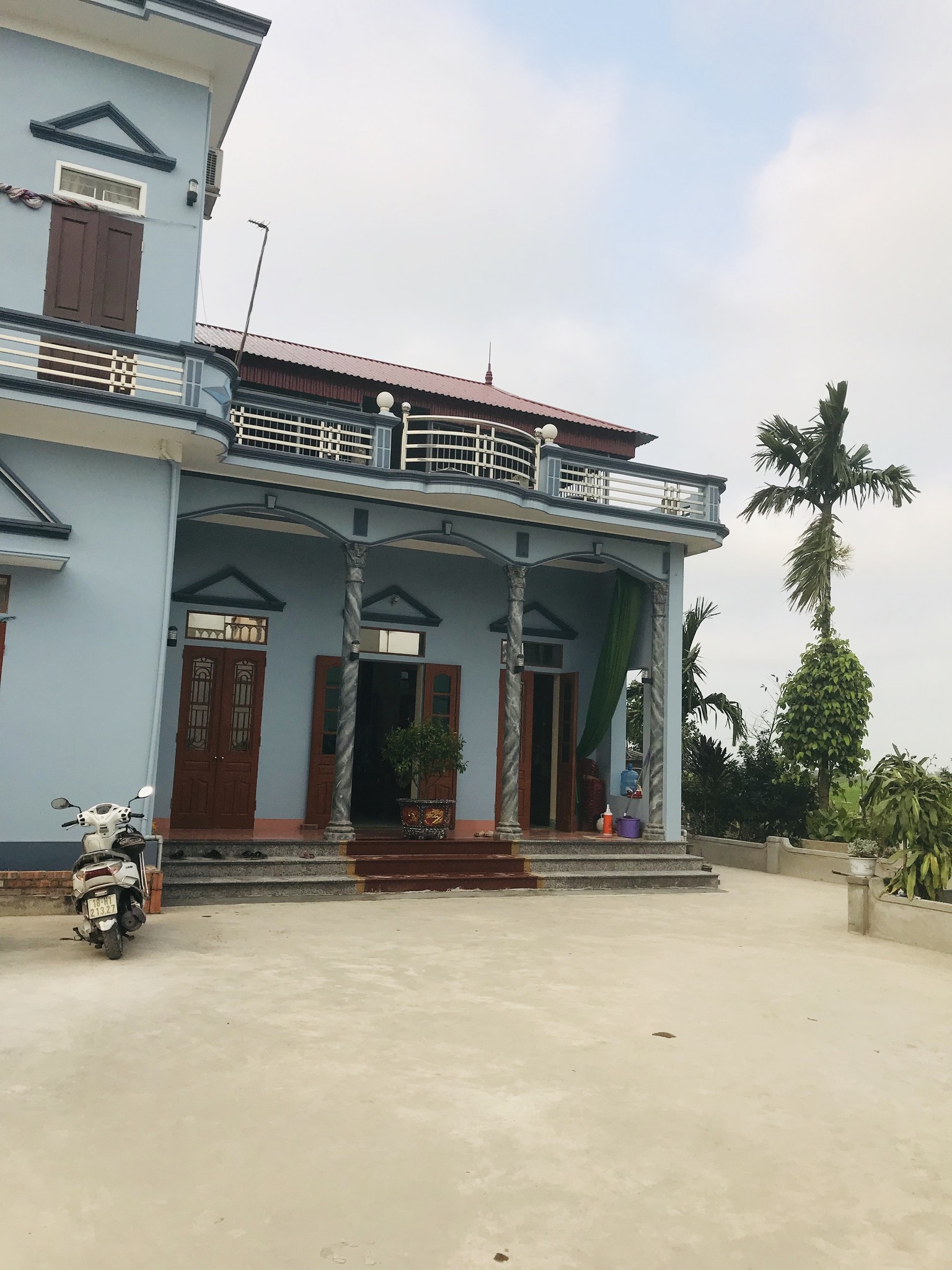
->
[{"xmin": 636, "ymin": 791, "xmax": 641, "ymax": 797}]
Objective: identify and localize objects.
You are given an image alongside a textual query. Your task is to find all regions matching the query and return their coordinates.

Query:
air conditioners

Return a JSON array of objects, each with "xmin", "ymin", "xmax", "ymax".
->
[{"xmin": 202, "ymin": 147, "xmax": 224, "ymax": 220}]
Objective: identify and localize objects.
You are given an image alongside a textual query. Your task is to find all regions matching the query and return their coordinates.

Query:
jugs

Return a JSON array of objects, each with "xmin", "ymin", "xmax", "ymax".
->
[{"xmin": 601, "ymin": 804, "xmax": 613, "ymax": 835}]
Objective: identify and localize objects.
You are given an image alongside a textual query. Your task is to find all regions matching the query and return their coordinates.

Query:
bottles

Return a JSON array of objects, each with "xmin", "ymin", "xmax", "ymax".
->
[{"xmin": 621, "ymin": 764, "xmax": 639, "ymax": 797}]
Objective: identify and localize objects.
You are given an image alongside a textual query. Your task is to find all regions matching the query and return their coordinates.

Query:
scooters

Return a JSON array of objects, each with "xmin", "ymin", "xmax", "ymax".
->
[{"xmin": 50, "ymin": 785, "xmax": 154, "ymax": 960}]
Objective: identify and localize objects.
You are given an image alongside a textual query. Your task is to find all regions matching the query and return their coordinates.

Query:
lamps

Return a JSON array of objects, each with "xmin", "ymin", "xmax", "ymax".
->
[
  {"xmin": 186, "ymin": 178, "xmax": 199, "ymax": 207},
  {"xmin": 350, "ymin": 640, "xmax": 360, "ymax": 661},
  {"xmin": 515, "ymin": 654, "xmax": 525, "ymax": 673},
  {"xmin": 167, "ymin": 626, "xmax": 178, "ymax": 647},
  {"xmin": 642, "ymin": 668, "xmax": 653, "ymax": 684}
]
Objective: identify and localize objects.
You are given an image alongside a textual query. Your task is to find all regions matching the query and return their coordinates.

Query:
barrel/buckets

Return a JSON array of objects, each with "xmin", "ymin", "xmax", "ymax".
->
[{"xmin": 615, "ymin": 816, "xmax": 641, "ymax": 838}]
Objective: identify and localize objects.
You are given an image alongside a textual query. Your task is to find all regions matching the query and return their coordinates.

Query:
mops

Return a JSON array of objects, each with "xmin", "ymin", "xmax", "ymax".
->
[{"xmin": 622, "ymin": 749, "xmax": 650, "ymax": 819}]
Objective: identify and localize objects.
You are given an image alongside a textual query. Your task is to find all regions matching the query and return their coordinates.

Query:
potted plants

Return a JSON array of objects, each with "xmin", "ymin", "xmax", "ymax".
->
[
  {"xmin": 847, "ymin": 838, "xmax": 880, "ymax": 878},
  {"xmin": 380, "ymin": 717, "xmax": 470, "ymax": 840}
]
